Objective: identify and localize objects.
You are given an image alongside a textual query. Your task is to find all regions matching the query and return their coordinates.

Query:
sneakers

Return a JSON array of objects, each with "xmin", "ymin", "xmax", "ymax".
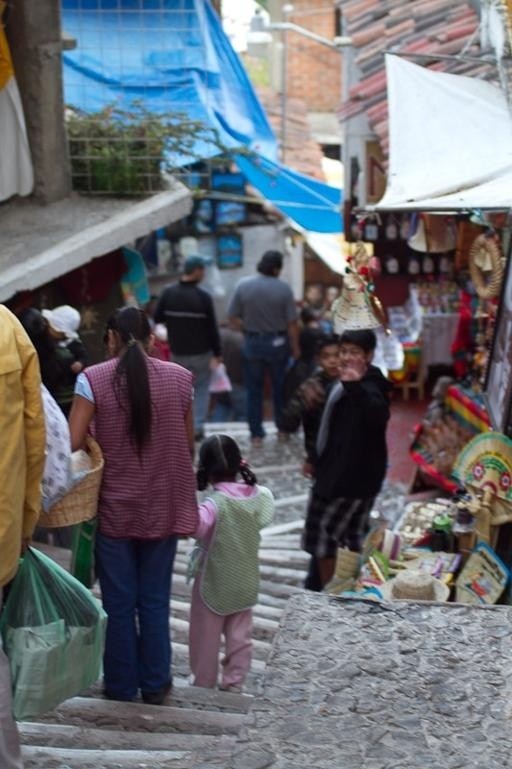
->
[
  {"xmin": 279, "ymin": 423, "xmax": 299, "ymax": 432},
  {"xmin": 250, "ymin": 429, "xmax": 266, "ymax": 438},
  {"xmin": 105, "ymin": 673, "xmax": 172, "ymax": 705}
]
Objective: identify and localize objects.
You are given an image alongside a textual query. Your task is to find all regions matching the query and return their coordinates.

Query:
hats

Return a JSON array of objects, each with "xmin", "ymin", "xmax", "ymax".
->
[
  {"xmin": 41, "ymin": 304, "xmax": 83, "ymax": 344},
  {"xmin": 379, "ymin": 569, "xmax": 451, "ymax": 601}
]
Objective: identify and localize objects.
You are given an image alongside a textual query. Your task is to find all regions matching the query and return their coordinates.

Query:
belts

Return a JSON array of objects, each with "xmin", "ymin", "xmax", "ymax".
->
[{"xmin": 244, "ymin": 330, "xmax": 286, "ymax": 338}]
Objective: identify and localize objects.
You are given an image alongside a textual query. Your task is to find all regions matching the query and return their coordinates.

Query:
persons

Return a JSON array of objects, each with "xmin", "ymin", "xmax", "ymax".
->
[
  {"xmin": 0, "ymin": 288, "xmax": 81, "ymax": 393},
  {"xmin": 276, "ymin": 332, "xmax": 345, "ymax": 594},
  {"xmin": 0, "ymin": 301, "xmax": 47, "ymax": 769},
  {"xmin": 298, "ymin": 330, "xmax": 396, "ymax": 589},
  {"xmin": 39, "ymin": 302, "xmax": 87, "ymax": 419},
  {"xmin": 186, "ymin": 432, "xmax": 276, "ymax": 694},
  {"xmin": 154, "ymin": 255, "xmax": 223, "ymax": 444},
  {"xmin": 68, "ymin": 305, "xmax": 202, "ymax": 707},
  {"xmin": 272, "ymin": 325, "xmax": 328, "ymax": 445},
  {"xmin": 226, "ymin": 249, "xmax": 303, "ymax": 446},
  {"xmin": 143, "ymin": 280, "xmax": 340, "ymax": 424}
]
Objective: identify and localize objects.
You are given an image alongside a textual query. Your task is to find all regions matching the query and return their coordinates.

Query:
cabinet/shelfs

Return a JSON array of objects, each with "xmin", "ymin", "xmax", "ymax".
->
[{"xmin": 389, "ymin": 281, "xmax": 468, "ymax": 404}]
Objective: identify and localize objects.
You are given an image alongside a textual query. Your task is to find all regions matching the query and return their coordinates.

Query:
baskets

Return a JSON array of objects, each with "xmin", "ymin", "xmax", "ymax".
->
[{"xmin": 37, "ymin": 434, "xmax": 106, "ymax": 530}]
